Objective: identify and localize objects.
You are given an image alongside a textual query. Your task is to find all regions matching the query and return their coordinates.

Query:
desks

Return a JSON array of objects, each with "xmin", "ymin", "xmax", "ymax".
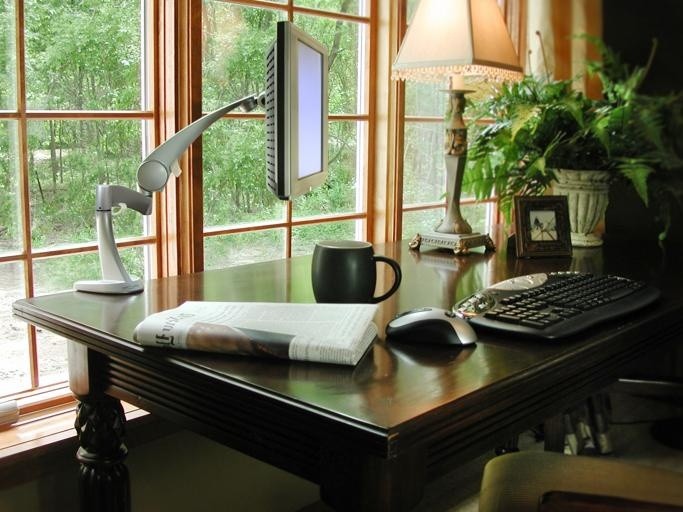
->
[{"xmin": 12, "ymin": 240, "xmax": 683, "ymax": 510}]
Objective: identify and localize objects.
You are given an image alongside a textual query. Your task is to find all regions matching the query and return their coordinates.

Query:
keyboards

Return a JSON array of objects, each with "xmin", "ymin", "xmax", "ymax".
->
[{"xmin": 452, "ymin": 271, "xmax": 664, "ymax": 341}]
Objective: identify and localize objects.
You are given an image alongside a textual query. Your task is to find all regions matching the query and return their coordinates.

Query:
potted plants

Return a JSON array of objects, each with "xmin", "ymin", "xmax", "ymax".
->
[{"xmin": 448, "ymin": 30, "xmax": 680, "ymax": 249}]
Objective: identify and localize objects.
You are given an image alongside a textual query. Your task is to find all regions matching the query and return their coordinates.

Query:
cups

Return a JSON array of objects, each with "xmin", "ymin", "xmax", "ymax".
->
[
  {"xmin": 318, "ymin": 331, "xmax": 399, "ymax": 386},
  {"xmin": 309, "ymin": 239, "xmax": 403, "ymax": 305}
]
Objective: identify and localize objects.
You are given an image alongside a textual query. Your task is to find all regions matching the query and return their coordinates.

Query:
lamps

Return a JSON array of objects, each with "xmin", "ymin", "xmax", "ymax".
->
[{"xmin": 389, "ymin": 0, "xmax": 524, "ymax": 260}]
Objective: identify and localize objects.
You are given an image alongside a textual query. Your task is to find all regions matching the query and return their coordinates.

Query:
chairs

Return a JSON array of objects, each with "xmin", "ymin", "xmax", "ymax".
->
[{"xmin": 473, "ymin": 375, "xmax": 682, "ymax": 511}]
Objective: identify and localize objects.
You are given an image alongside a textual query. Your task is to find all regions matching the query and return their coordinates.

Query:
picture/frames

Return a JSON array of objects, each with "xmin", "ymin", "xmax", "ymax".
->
[{"xmin": 512, "ymin": 194, "xmax": 574, "ymax": 258}]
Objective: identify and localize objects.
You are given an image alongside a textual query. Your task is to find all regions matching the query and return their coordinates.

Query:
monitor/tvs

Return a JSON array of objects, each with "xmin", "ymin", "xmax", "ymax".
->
[{"xmin": 74, "ymin": 21, "xmax": 328, "ymax": 294}]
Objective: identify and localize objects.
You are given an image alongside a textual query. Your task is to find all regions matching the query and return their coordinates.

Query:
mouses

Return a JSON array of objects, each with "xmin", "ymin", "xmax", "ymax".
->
[{"xmin": 385, "ymin": 306, "xmax": 477, "ymax": 347}]
